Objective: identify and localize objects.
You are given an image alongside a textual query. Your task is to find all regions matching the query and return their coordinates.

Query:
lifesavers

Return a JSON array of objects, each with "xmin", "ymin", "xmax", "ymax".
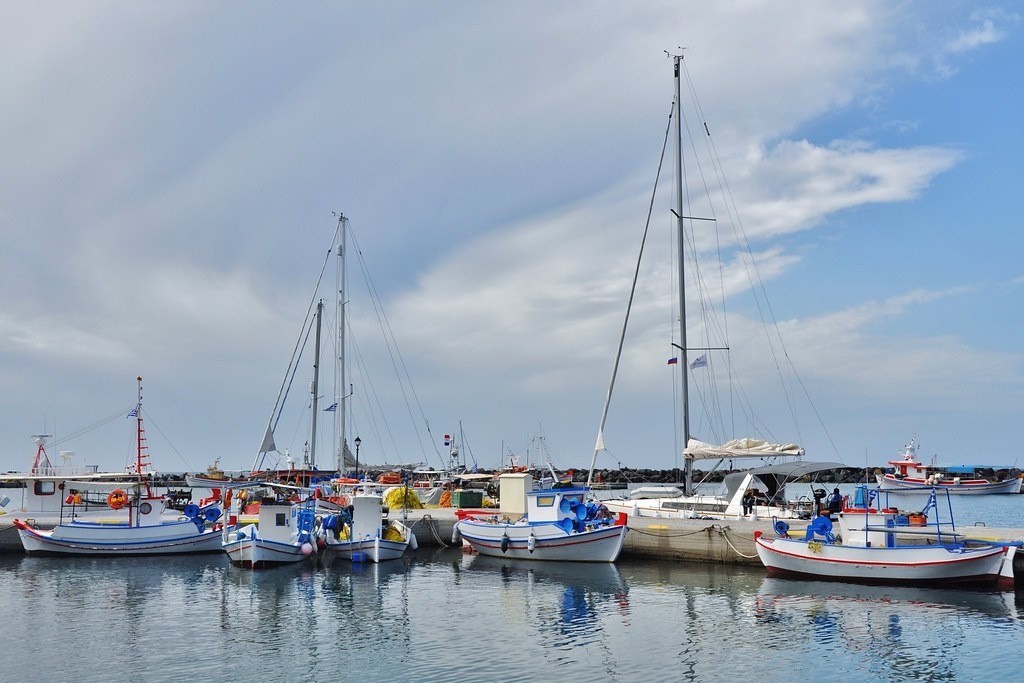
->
[
  {"xmin": 107, "ymin": 490, "xmax": 127, "ymax": 509},
  {"xmin": 225, "ymin": 488, "xmax": 232, "ymax": 508}
]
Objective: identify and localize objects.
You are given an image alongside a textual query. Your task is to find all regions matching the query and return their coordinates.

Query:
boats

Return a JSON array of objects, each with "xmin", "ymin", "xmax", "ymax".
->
[
  {"xmin": 14, "ymin": 480, "xmax": 237, "ymax": 553},
  {"xmin": 875, "ymin": 432, "xmax": 1024, "ymax": 494},
  {"xmin": 753, "ymin": 446, "xmax": 1024, "ymax": 618},
  {"xmin": 322, "ymin": 485, "xmax": 419, "ymax": 564},
  {"xmin": 0, "ymin": 476, "xmax": 186, "ymax": 548},
  {"xmin": 451, "ymin": 473, "xmax": 627, "ymax": 563},
  {"xmin": 221, "ymin": 482, "xmax": 323, "ymax": 570}
]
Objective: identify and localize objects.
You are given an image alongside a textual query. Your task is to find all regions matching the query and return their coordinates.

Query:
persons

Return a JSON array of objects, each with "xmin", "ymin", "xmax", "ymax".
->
[{"xmin": 828, "ymin": 488, "xmax": 842, "ymax": 512}]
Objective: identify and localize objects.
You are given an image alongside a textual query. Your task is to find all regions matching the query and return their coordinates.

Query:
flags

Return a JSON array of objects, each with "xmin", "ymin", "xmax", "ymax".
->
[
  {"xmin": 127, "ymin": 405, "xmax": 140, "ymax": 419},
  {"xmin": 668, "ymin": 358, "xmax": 677, "ymax": 365},
  {"xmin": 323, "ymin": 402, "xmax": 339, "ymax": 412},
  {"xmin": 689, "ymin": 354, "xmax": 708, "ymax": 369},
  {"xmin": 471, "ymin": 463, "xmax": 478, "ymax": 473}
]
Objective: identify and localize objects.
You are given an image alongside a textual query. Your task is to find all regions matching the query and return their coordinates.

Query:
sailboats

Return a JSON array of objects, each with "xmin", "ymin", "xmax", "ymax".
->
[{"xmin": 31, "ymin": 44, "xmax": 940, "ymax": 549}]
